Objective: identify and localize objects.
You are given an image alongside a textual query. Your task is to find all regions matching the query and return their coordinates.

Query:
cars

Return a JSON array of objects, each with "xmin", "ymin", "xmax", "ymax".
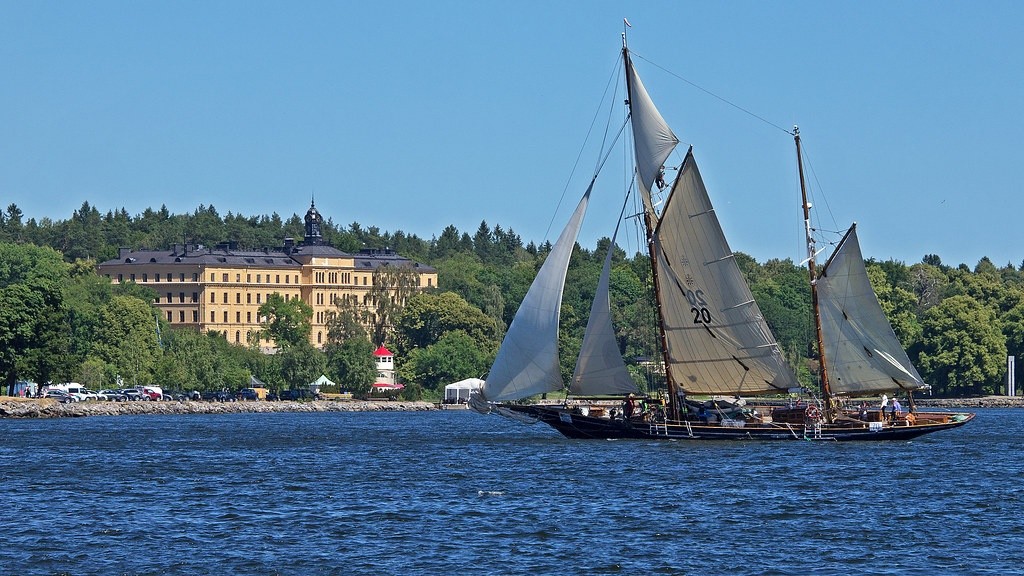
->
[{"xmin": 44, "ymin": 385, "xmax": 321, "ymax": 405}]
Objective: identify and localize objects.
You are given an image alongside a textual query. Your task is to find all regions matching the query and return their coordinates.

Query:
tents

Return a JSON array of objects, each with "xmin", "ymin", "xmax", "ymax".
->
[{"xmin": 308, "ymin": 375, "xmax": 335, "ymax": 389}]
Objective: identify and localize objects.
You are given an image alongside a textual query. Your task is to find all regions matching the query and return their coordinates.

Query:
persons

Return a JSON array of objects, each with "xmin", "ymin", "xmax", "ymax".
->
[
  {"xmin": 769, "ymin": 406, "xmax": 773, "ymax": 413},
  {"xmin": 892, "ymin": 398, "xmax": 901, "ymax": 412},
  {"xmin": 696, "ymin": 404, "xmax": 712, "ymax": 421},
  {"xmin": 858, "ymin": 404, "xmax": 867, "ymax": 421},
  {"xmin": 798, "ymin": 398, "xmax": 801, "ymax": 404},
  {"xmin": 655, "ymin": 166, "xmax": 665, "ymax": 192},
  {"xmin": 751, "ymin": 407, "xmax": 756, "ymax": 414},
  {"xmin": 880, "ymin": 393, "xmax": 888, "ymax": 420}
]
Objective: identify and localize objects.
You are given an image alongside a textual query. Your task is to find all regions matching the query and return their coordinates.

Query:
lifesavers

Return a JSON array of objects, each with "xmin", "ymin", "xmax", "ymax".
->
[{"xmin": 806, "ymin": 406, "xmax": 819, "ymax": 419}]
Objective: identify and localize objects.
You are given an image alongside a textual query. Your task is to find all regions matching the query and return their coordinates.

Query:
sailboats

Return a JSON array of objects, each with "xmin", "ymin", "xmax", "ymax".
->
[{"xmin": 467, "ymin": 18, "xmax": 976, "ymax": 442}]
